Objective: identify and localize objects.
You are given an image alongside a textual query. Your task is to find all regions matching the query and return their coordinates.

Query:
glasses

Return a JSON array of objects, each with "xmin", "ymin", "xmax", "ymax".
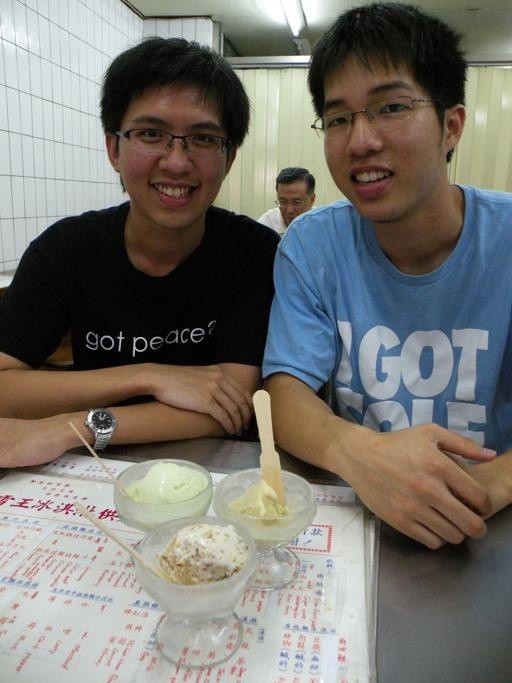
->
[
  {"xmin": 110, "ymin": 125, "xmax": 233, "ymax": 156},
  {"xmin": 310, "ymin": 95, "xmax": 442, "ymax": 141},
  {"xmin": 275, "ymin": 192, "xmax": 313, "ymax": 208}
]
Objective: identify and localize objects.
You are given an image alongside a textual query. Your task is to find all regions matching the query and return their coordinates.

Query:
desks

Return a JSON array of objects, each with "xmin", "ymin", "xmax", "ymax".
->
[{"xmin": 0, "ymin": 439, "xmax": 512, "ymax": 683}]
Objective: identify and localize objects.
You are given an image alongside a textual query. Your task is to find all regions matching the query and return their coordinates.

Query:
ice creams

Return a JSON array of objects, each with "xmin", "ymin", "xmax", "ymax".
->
[
  {"xmin": 230, "ymin": 480, "xmax": 289, "ymax": 518},
  {"xmin": 159, "ymin": 523, "xmax": 247, "ymax": 585},
  {"xmin": 124, "ymin": 463, "xmax": 208, "ymax": 502}
]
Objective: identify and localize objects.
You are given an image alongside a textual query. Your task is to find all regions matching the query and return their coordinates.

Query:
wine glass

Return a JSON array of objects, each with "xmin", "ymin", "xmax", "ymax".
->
[
  {"xmin": 213, "ymin": 464, "xmax": 316, "ymax": 591},
  {"xmin": 112, "ymin": 459, "xmax": 213, "ymax": 535},
  {"xmin": 133, "ymin": 514, "xmax": 257, "ymax": 669}
]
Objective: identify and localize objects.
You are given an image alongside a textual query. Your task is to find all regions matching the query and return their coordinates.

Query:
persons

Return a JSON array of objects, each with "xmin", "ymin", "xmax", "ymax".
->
[
  {"xmin": 1, "ymin": 32, "xmax": 284, "ymax": 473},
  {"xmin": 254, "ymin": 0, "xmax": 511, "ymax": 555},
  {"xmin": 253, "ymin": 165, "xmax": 324, "ymax": 239}
]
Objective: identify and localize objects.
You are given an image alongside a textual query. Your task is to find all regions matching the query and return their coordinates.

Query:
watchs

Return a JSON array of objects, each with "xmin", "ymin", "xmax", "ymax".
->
[{"xmin": 83, "ymin": 405, "xmax": 117, "ymax": 454}]
